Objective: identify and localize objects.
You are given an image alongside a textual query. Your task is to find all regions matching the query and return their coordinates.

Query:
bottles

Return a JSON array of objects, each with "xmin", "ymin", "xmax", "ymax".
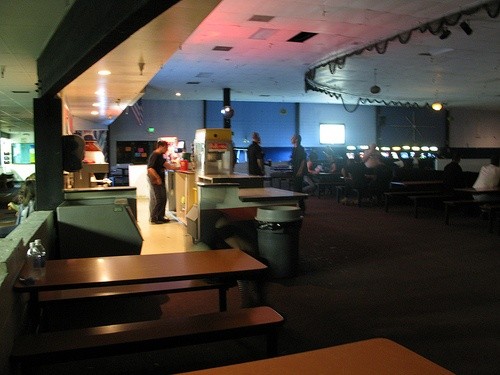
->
[
  {"xmin": 32, "ymin": 239, "xmax": 47, "ymax": 276},
  {"xmin": 25, "ymin": 242, "xmax": 42, "ymax": 280}
]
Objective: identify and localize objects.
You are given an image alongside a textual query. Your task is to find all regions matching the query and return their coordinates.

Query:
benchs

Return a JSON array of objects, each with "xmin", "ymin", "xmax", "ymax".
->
[
  {"xmin": 13, "ymin": 306, "xmax": 285, "ymax": 375},
  {"xmin": 27, "ymin": 278, "xmax": 227, "ymax": 322}
]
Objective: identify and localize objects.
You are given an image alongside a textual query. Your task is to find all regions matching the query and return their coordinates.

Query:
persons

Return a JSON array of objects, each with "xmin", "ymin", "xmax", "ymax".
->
[
  {"xmin": 472, "ymin": 152, "xmax": 499, "ymax": 210},
  {"xmin": 247, "ymin": 132, "xmax": 265, "ymax": 177},
  {"xmin": 147, "ymin": 140, "xmax": 174, "ymax": 225},
  {"xmin": 288, "ymin": 135, "xmax": 309, "ymax": 216},
  {"xmin": 300, "ymin": 152, "xmax": 321, "ymax": 194},
  {"xmin": 360, "ymin": 142, "xmax": 387, "ymax": 190}
]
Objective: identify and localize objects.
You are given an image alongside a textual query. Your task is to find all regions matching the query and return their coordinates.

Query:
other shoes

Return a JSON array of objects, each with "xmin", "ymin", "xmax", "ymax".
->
[{"xmin": 150, "ymin": 219, "xmax": 170, "ymax": 225}]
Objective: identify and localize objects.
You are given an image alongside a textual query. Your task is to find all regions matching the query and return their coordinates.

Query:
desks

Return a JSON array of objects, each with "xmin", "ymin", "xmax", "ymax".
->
[
  {"xmin": 235, "ymin": 187, "xmax": 310, "ymax": 216},
  {"xmin": 12, "ymin": 249, "xmax": 269, "ymax": 323},
  {"xmin": 264, "ymin": 160, "xmax": 500, "ymax": 219},
  {"xmin": 173, "ymin": 336, "xmax": 456, "ymax": 375}
]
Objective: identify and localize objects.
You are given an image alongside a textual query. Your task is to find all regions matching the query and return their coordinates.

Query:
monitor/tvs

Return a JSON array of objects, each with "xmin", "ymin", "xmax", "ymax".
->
[{"xmin": 11, "ymin": 142, "xmax": 36, "ymax": 165}]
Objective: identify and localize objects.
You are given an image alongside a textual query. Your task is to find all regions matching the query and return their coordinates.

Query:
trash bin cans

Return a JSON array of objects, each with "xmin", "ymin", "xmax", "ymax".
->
[{"xmin": 255, "ymin": 204, "xmax": 303, "ymax": 284}]
